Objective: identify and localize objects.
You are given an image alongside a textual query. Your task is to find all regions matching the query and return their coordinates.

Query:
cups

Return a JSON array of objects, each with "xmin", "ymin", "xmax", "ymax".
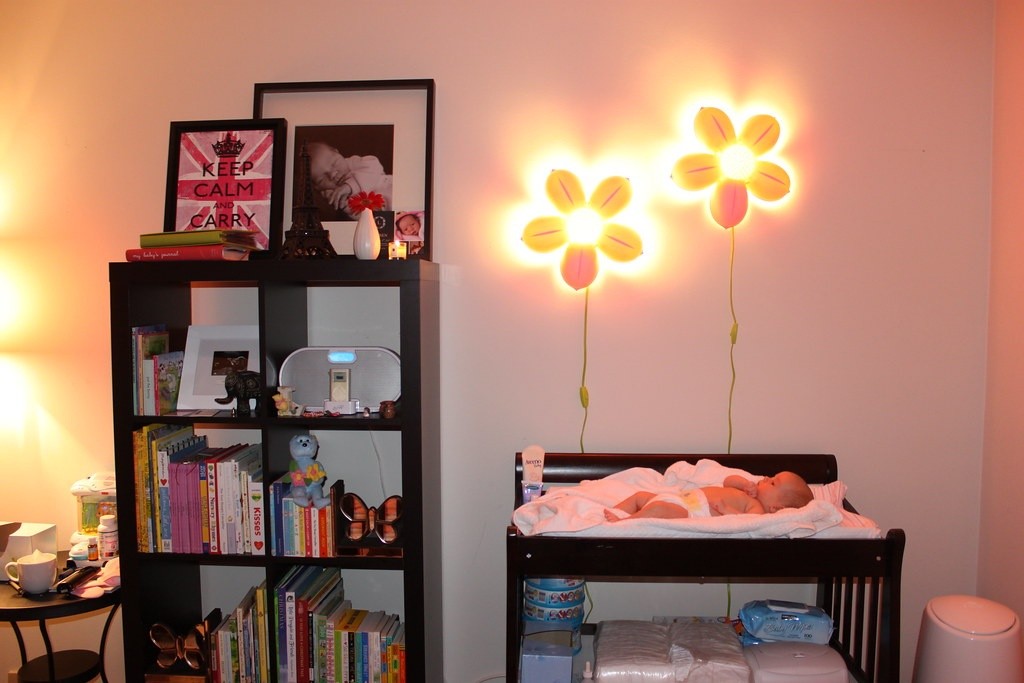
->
[{"xmin": 4, "ymin": 552, "xmax": 58, "ymax": 594}]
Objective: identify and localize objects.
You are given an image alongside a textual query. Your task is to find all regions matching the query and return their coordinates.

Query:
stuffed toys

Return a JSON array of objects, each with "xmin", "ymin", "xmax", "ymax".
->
[{"xmin": 289, "ymin": 435, "xmax": 331, "ymax": 509}]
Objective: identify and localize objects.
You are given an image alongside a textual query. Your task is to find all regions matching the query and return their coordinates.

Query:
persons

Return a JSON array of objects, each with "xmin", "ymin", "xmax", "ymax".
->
[
  {"xmin": 307, "ymin": 143, "xmax": 393, "ymax": 221},
  {"xmin": 395, "ymin": 211, "xmax": 424, "ymax": 240},
  {"xmin": 605, "ymin": 470, "xmax": 814, "ymax": 524}
]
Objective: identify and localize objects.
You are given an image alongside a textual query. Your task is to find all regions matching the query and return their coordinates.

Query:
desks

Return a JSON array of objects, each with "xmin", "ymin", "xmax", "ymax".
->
[{"xmin": 0, "ymin": 550, "xmax": 121, "ymax": 683}]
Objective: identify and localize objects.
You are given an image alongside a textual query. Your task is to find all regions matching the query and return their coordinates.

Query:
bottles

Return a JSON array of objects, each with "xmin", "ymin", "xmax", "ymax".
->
[
  {"xmin": 87, "ymin": 537, "xmax": 98, "ymax": 561},
  {"xmin": 97, "ymin": 514, "xmax": 120, "ymax": 560}
]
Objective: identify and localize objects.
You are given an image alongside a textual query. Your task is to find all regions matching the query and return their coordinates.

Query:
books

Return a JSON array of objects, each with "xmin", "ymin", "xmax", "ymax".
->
[
  {"xmin": 125, "ymin": 246, "xmax": 253, "ymax": 261},
  {"xmin": 274, "ymin": 566, "xmax": 406, "ymax": 683},
  {"xmin": 132, "ymin": 423, "xmax": 265, "ymax": 555},
  {"xmin": 204, "ymin": 579, "xmax": 271, "ymax": 683},
  {"xmin": 268, "ymin": 473, "xmax": 345, "ymax": 558},
  {"xmin": 140, "ymin": 229, "xmax": 259, "ymax": 249},
  {"xmin": 132, "ymin": 324, "xmax": 184, "ymax": 416}
]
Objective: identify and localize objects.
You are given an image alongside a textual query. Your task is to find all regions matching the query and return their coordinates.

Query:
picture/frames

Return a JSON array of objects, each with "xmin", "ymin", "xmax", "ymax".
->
[
  {"xmin": 163, "ymin": 118, "xmax": 287, "ymax": 258},
  {"xmin": 254, "ymin": 79, "xmax": 437, "ymax": 261},
  {"xmin": 176, "ymin": 324, "xmax": 260, "ymax": 410}
]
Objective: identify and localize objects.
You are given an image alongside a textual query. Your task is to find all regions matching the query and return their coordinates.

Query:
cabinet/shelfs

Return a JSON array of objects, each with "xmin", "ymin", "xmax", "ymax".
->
[
  {"xmin": 505, "ymin": 451, "xmax": 906, "ymax": 683},
  {"xmin": 109, "ymin": 260, "xmax": 445, "ymax": 683}
]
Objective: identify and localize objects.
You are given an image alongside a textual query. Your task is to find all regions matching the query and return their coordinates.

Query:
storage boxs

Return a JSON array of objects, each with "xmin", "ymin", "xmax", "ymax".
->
[{"xmin": 0, "ymin": 521, "xmax": 56, "ymax": 584}]
